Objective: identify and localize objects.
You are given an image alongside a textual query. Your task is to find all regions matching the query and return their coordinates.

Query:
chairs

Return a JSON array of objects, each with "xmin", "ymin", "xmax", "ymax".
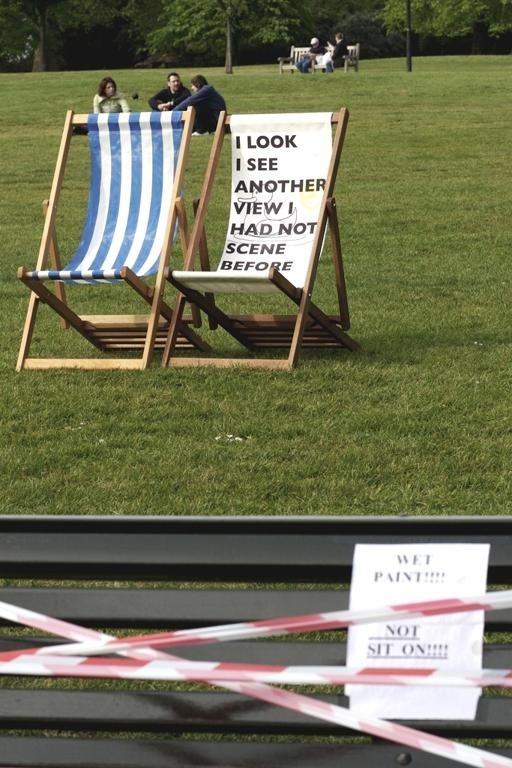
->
[
  {"xmin": 158, "ymin": 107, "xmax": 361, "ymax": 371},
  {"xmin": 14, "ymin": 106, "xmax": 210, "ymax": 372}
]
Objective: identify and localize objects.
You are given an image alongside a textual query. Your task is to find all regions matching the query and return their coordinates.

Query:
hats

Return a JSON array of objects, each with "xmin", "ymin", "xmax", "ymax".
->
[{"xmin": 310, "ymin": 37, "xmax": 319, "ymax": 45}]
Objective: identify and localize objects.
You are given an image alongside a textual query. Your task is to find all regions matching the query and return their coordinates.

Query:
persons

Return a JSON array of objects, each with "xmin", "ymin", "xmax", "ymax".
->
[
  {"xmin": 171, "ymin": 74, "xmax": 228, "ymax": 136},
  {"xmin": 148, "ymin": 71, "xmax": 191, "ymax": 112},
  {"xmin": 322, "ymin": 32, "xmax": 350, "ymax": 72},
  {"xmin": 295, "ymin": 37, "xmax": 328, "ymax": 73},
  {"xmin": 92, "ymin": 75, "xmax": 132, "ymax": 113}
]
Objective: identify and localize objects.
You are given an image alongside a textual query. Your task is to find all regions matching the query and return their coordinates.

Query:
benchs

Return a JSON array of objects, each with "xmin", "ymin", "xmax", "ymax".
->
[{"xmin": 278, "ymin": 41, "xmax": 361, "ymax": 74}]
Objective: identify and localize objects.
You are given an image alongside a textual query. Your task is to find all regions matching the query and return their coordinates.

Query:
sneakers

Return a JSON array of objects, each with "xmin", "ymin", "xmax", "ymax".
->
[{"xmin": 190, "ymin": 131, "xmax": 210, "ymax": 136}]
